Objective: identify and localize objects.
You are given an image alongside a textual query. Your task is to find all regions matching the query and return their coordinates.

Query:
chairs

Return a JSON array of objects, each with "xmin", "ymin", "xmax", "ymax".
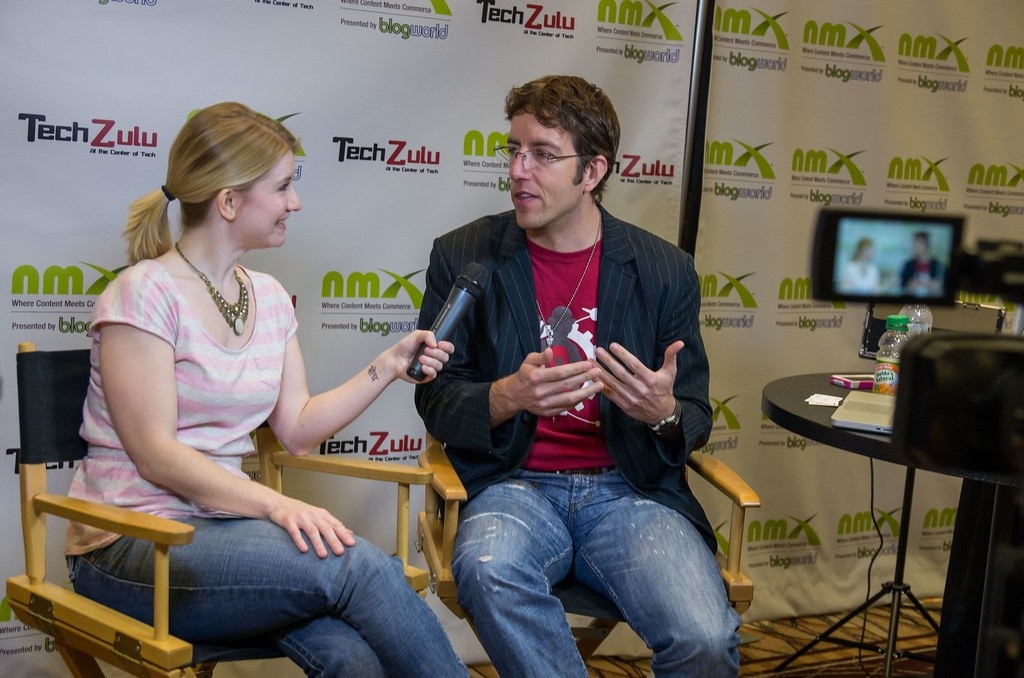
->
[
  {"xmin": 415, "ymin": 424, "xmax": 762, "ymax": 664},
  {"xmin": 6, "ymin": 343, "xmax": 437, "ymax": 678}
]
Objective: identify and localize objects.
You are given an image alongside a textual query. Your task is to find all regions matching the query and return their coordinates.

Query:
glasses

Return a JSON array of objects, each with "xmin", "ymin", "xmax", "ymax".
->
[{"xmin": 493, "ymin": 143, "xmax": 588, "ymax": 171}]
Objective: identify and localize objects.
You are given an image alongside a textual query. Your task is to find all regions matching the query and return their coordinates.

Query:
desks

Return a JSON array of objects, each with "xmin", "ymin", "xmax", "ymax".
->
[{"xmin": 759, "ymin": 369, "xmax": 1024, "ymax": 677}]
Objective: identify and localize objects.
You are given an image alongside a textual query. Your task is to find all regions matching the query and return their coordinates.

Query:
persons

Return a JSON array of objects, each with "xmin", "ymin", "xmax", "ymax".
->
[
  {"xmin": 66, "ymin": 102, "xmax": 467, "ymax": 678},
  {"xmin": 898, "ymin": 233, "xmax": 943, "ymax": 298},
  {"xmin": 842, "ymin": 236, "xmax": 889, "ymax": 293},
  {"xmin": 411, "ymin": 76, "xmax": 743, "ymax": 678}
]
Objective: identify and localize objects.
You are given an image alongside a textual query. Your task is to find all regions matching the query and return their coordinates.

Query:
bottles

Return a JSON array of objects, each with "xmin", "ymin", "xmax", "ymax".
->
[
  {"xmin": 898, "ymin": 304, "xmax": 933, "ymax": 338},
  {"xmin": 872, "ymin": 315, "xmax": 909, "ymax": 396}
]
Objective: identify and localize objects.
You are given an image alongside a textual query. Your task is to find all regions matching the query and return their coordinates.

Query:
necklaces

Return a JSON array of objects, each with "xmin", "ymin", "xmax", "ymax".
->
[
  {"xmin": 530, "ymin": 213, "xmax": 602, "ymax": 345},
  {"xmin": 174, "ymin": 242, "xmax": 250, "ymax": 337}
]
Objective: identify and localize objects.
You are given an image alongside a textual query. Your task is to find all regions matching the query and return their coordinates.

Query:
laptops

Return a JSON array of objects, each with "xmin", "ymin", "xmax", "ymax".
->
[{"xmin": 830, "ymin": 391, "xmax": 896, "ymax": 435}]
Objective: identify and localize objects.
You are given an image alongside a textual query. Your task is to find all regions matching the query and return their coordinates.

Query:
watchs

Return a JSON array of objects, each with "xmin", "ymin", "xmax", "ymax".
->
[{"xmin": 646, "ymin": 397, "xmax": 683, "ymax": 436}]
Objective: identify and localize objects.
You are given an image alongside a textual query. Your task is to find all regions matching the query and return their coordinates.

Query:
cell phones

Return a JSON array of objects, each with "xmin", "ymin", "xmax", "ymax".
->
[{"xmin": 830, "ymin": 374, "xmax": 875, "ymax": 389}]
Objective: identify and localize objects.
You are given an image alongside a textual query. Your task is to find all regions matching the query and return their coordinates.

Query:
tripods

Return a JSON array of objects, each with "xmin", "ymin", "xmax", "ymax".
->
[{"xmin": 771, "ymin": 468, "xmax": 940, "ymax": 678}]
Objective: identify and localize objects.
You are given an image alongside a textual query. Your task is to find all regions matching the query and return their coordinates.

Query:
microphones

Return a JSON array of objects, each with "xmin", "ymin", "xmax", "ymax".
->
[{"xmin": 405, "ymin": 261, "xmax": 488, "ymax": 382}]
